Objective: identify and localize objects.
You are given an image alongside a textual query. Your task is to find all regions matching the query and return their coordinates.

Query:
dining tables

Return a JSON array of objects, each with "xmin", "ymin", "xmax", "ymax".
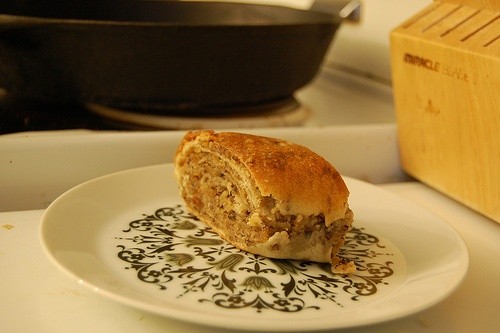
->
[{"xmin": 1, "ymin": 179, "xmax": 500, "ymax": 332}]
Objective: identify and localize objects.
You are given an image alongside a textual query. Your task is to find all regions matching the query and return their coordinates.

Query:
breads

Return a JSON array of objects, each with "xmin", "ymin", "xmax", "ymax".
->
[{"xmin": 173, "ymin": 128, "xmax": 357, "ymax": 274}]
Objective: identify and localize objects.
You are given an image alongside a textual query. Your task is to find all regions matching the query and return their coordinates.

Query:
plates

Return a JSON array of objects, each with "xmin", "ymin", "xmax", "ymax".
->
[
  {"xmin": 39, "ymin": 162, "xmax": 469, "ymax": 331},
  {"xmin": 91, "ymin": 102, "xmax": 305, "ymax": 127}
]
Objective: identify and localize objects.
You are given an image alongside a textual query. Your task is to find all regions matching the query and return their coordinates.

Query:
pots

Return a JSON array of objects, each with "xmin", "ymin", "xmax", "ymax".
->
[{"xmin": 0, "ymin": 1, "xmax": 344, "ymax": 105}]
